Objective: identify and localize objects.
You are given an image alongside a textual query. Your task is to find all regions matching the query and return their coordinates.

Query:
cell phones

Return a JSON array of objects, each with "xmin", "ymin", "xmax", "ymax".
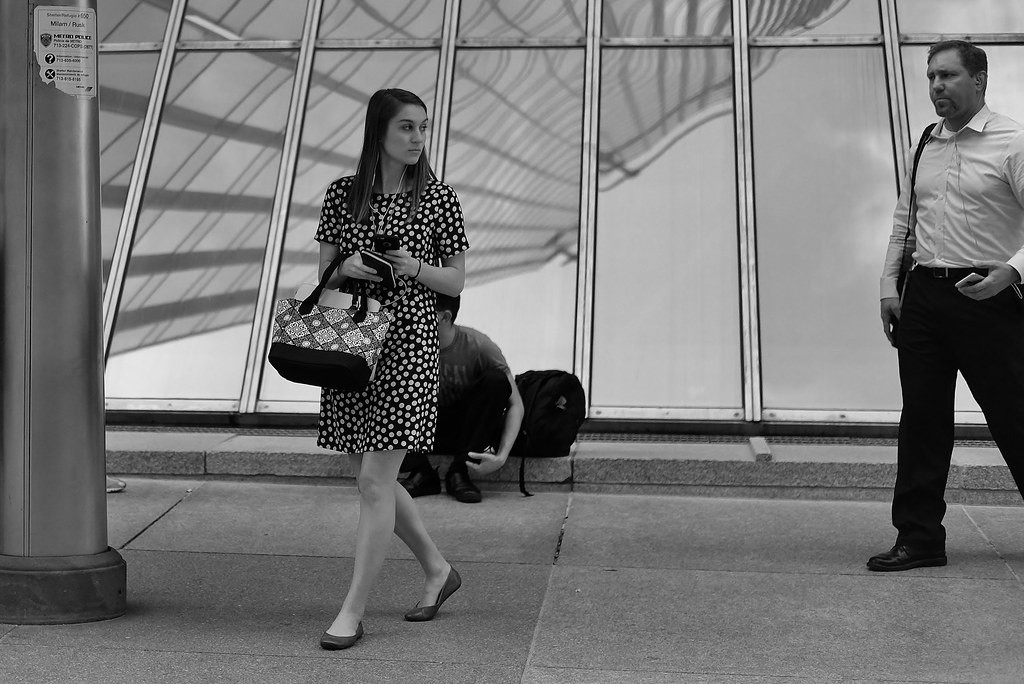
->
[
  {"xmin": 955, "ymin": 273, "xmax": 985, "ymax": 288},
  {"xmin": 373, "ymin": 234, "xmax": 400, "ymax": 254}
]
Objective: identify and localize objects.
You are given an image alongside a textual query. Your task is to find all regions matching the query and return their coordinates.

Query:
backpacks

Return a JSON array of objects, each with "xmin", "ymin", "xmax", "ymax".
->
[{"xmin": 493, "ymin": 370, "xmax": 586, "ymax": 496}]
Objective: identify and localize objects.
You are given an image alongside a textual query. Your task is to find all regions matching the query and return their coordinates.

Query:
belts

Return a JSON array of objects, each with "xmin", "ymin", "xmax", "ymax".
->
[{"xmin": 913, "ymin": 265, "xmax": 989, "ymax": 279}]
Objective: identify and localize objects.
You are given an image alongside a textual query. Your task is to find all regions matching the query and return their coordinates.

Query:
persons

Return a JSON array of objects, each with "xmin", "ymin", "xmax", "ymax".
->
[
  {"xmin": 864, "ymin": 40, "xmax": 1024, "ymax": 572},
  {"xmin": 265, "ymin": 88, "xmax": 469, "ymax": 650},
  {"xmin": 399, "ymin": 288, "xmax": 526, "ymax": 503}
]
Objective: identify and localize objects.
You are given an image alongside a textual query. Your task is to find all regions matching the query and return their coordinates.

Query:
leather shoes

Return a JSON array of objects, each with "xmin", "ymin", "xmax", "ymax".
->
[
  {"xmin": 867, "ymin": 545, "xmax": 947, "ymax": 572},
  {"xmin": 445, "ymin": 473, "xmax": 482, "ymax": 503},
  {"xmin": 400, "ymin": 466, "xmax": 442, "ymax": 498}
]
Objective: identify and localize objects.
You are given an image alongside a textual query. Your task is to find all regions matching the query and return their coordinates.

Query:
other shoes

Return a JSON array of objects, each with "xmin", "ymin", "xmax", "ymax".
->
[
  {"xmin": 405, "ymin": 565, "xmax": 461, "ymax": 621},
  {"xmin": 321, "ymin": 621, "xmax": 363, "ymax": 649},
  {"xmin": 106, "ymin": 474, "xmax": 126, "ymax": 493}
]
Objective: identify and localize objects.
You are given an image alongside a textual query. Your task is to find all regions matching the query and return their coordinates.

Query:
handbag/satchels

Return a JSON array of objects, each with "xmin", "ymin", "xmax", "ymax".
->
[{"xmin": 268, "ymin": 254, "xmax": 394, "ymax": 392}]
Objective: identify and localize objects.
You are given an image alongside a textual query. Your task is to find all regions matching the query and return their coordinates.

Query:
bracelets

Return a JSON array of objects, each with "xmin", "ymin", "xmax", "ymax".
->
[
  {"xmin": 409, "ymin": 259, "xmax": 421, "ymax": 279},
  {"xmin": 337, "ymin": 260, "xmax": 344, "ymax": 278}
]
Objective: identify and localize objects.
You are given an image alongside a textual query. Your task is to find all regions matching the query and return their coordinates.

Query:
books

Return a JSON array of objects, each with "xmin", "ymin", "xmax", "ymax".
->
[{"xmin": 359, "ymin": 248, "xmax": 397, "ymax": 290}]
